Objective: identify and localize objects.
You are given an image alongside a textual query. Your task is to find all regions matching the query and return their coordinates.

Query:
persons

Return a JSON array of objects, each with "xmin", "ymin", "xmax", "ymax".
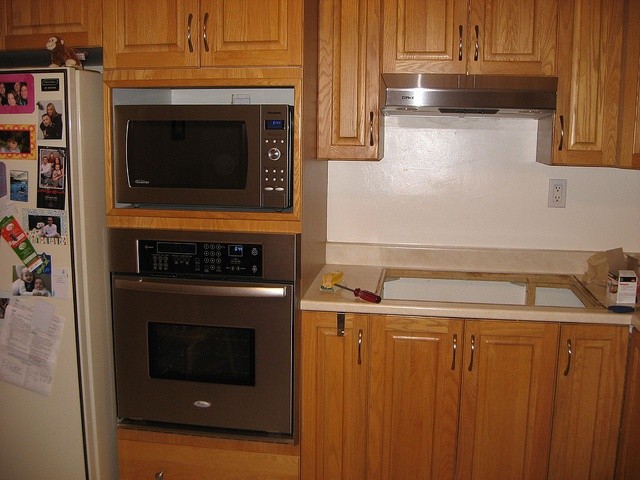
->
[
  {"xmin": 47, "ymin": 152, "xmax": 55, "ymax": 163},
  {"xmin": 20, "ymin": 83, "xmax": 27, "ymax": 100},
  {"xmin": 12, "ymin": 268, "xmax": 42, "ymax": 296},
  {"xmin": 51, "ymin": 157, "xmax": 63, "ymax": 188},
  {"xmin": 1, "ymin": 134, "xmax": 21, "ymax": 153},
  {"xmin": 40, "ymin": 157, "xmax": 52, "ymax": 184},
  {"xmin": 40, "ymin": 103, "xmax": 62, "ymax": 137},
  {"xmin": 42, "ymin": 217, "xmax": 61, "ymax": 238},
  {"xmin": 52, "ymin": 165, "xmax": 61, "ymax": 187},
  {"xmin": 0, "ymin": 83, "xmax": 6, "ymax": 95},
  {"xmin": 7, "ymin": 90, "xmax": 20, "ymax": 106},
  {"xmin": 42, "ymin": 114, "xmax": 62, "ymax": 139},
  {"xmin": 32, "ymin": 277, "xmax": 51, "ymax": 296},
  {"xmin": 14, "ymin": 82, "xmax": 20, "ymax": 91}
]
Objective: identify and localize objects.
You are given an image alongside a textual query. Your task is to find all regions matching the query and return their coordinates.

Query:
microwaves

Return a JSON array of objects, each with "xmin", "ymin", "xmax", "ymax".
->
[{"xmin": 114, "ymin": 104, "xmax": 294, "ymax": 212}]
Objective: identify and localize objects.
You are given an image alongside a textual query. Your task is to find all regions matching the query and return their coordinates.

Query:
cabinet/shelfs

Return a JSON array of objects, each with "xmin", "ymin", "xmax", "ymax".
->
[
  {"xmin": 615, "ymin": 0, "xmax": 639, "ymax": 170},
  {"xmin": 118, "ymin": 422, "xmax": 301, "ymax": 480},
  {"xmin": 0, "ymin": 0, "xmax": 102, "ymax": 51},
  {"xmin": 616, "ymin": 327, "xmax": 640, "ymax": 480},
  {"xmin": 103, "ymin": 1, "xmax": 328, "ymax": 234},
  {"xmin": 381, "ymin": 0, "xmax": 557, "ymax": 93},
  {"xmin": 300, "ymin": 310, "xmax": 369, "ymax": 480},
  {"xmin": 548, "ymin": 323, "xmax": 629, "ymax": 480},
  {"xmin": 316, "ymin": 0, "xmax": 386, "ymax": 162},
  {"xmin": 367, "ymin": 314, "xmax": 560, "ymax": 480},
  {"xmin": 536, "ymin": 0, "xmax": 618, "ymax": 167}
]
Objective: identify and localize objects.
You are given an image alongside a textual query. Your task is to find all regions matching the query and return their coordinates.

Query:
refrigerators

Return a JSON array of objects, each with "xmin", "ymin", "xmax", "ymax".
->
[{"xmin": 0, "ymin": 67, "xmax": 117, "ymax": 479}]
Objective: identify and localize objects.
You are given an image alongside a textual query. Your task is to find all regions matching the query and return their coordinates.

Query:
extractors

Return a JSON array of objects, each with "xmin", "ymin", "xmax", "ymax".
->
[{"xmin": 381, "ymin": 76, "xmax": 559, "ymax": 117}]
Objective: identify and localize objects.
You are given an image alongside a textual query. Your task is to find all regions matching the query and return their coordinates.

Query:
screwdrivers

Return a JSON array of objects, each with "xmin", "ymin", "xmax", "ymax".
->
[{"xmin": 334, "ymin": 283, "xmax": 381, "ymax": 303}]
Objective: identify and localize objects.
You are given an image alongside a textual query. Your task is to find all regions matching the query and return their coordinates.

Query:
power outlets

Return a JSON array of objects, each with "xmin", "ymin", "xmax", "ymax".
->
[{"xmin": 547, "ymin": 179, "xmax": 566, "ymax": 208}]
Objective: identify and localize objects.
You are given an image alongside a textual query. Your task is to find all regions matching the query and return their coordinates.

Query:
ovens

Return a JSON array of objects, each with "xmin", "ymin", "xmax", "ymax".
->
[{"xmin": 111, "ymin": 228, "xmax": 300, "ymax": 437}]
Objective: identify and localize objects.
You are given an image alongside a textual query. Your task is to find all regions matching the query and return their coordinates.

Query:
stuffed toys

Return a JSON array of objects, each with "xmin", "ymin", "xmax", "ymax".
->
[{"xmin": 46, "ymin": 36, "xmax": 81, "ymax": 67}]
